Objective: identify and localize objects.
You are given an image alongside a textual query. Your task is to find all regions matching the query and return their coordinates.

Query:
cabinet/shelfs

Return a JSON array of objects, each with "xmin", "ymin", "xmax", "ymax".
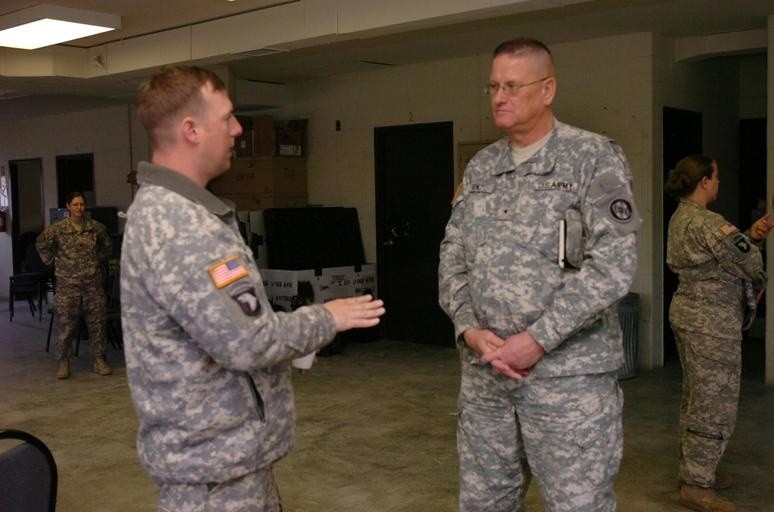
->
[{"xmin": 208, "ymin": 156, "xmax": 309, "ymax": 213}]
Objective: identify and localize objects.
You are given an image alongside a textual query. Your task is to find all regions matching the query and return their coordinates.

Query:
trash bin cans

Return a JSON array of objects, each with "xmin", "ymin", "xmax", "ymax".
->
[{"xmin": 615, "ymin": 290, "xmax": 641, "ymax": 381}]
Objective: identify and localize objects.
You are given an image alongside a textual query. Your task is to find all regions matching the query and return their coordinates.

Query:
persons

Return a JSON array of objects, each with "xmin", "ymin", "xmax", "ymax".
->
[
  {"xmin": 438, "ymin": 38, "xmax": 644, "ymax": 512},
  {"xmin": 36, "ymin": 189, "xmax": 114, "ymax": 380},
  {"xmin": 664, "ymin": 154, "xmax": 774, "ymax": 512},
  {"xmin": 120, "ymin": 63, "xmax": 388, "ymax": 512}
]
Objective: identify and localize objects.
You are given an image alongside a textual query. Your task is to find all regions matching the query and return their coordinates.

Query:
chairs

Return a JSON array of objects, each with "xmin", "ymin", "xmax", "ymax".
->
[
  {"xmin": 0, "ymin": 428, "xmax": 58, "ymax": 511},
  {"xmin": 2, "ymin": 231, "xmax": 124, "ymax": 358}
]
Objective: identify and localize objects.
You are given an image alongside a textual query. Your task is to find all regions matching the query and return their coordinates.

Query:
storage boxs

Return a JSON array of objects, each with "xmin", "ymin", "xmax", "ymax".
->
[
  {"xmin": 278, "ymin": 143, "xmax": 302, "ymax": 157},
  {"xmin": 257, "ymin": 260, "xmax": 379, "ymax": 313}
]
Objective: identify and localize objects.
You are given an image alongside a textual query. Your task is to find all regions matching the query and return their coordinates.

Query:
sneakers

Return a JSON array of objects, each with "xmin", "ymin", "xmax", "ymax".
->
[
  {"xmin": 92, "ymin": 355, "xmax": 112, "ymax": 375},
  {"xmin": 56, "ymin": 359, "xmax": 72, "ymax": 379},
  {"xmin": 679, "ymin": 483, "xmax": 736, "ymax": 512},
  {"xmin": 677, "ymin": 474, "xmax": 734, "ymax": 489}
]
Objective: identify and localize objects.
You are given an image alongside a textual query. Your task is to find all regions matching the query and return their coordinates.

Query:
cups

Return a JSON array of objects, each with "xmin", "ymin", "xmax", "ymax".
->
[{"xmin": 290, "ymin": 348, "xmax": 316, "ymax": 370}]
